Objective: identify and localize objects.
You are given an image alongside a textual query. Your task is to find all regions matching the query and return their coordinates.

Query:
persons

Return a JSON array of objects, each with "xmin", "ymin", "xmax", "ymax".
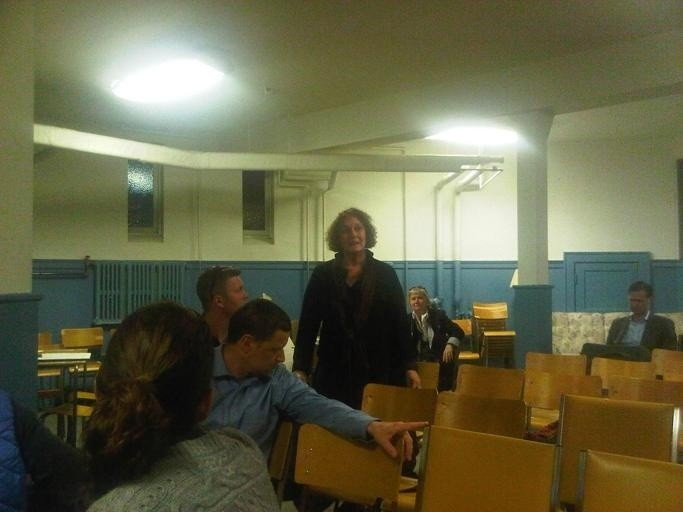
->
[
  {"xmin": 195, "ymin": 300, "xmax": 430, "ymax": 471},
  {"xmin": 1, "ymin": 391, "xmax": 88, "ymax": 512},
  {"xmin": 294, "ymin": 208, "xmax": 423, "ymax": 511},
  {"xmin": 195, "ymin": 263, "xmax": 247, "ymax": 343},
  {"xmin": 606, "ymin": 282, "xmax": 678, "ymax": 354},
  {"xmin": 406, "ymin": 285, "xmax": 465, "ymax": 392},
  {"xmin": 69, "ymin": 302, "xmax": 280, "ymax": 512}
]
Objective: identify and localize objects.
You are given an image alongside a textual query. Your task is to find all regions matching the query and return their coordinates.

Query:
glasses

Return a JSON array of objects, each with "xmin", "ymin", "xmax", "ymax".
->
[{"xmin": 210, "ymin": 265, "xmax": 223, "ymax": 294}]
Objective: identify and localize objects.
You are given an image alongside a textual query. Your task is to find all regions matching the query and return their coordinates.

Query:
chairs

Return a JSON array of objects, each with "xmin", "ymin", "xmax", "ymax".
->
[{"xmin": 36, "ymin": 327, "xmax": 119, "ymax": 448}]
